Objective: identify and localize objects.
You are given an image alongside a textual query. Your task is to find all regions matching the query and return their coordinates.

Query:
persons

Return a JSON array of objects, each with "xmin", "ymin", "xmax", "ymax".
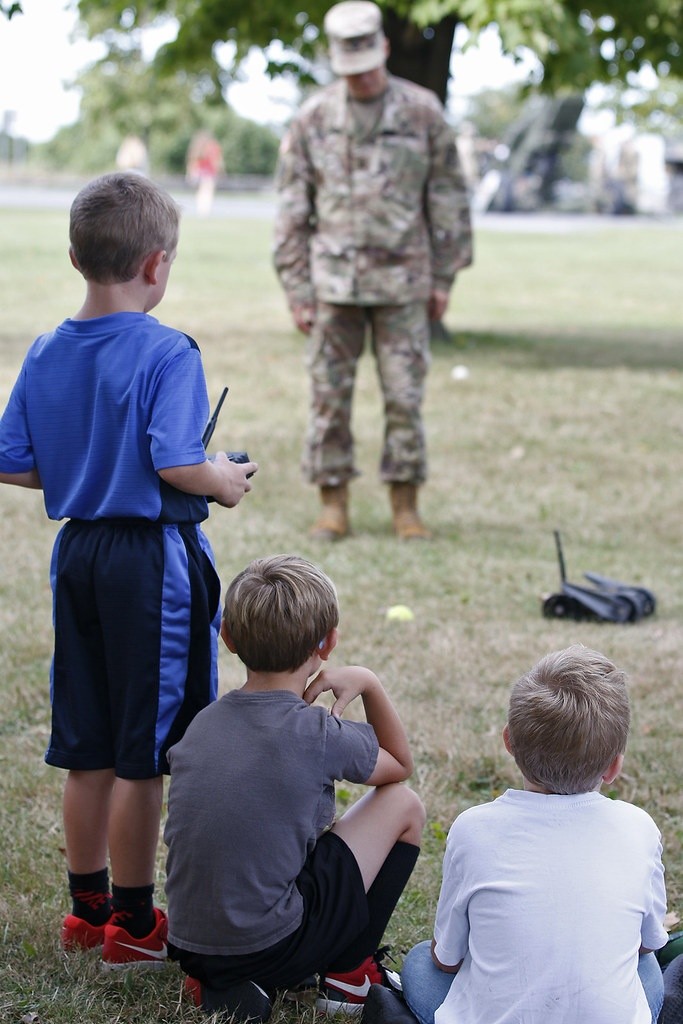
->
[
  {"xmin": 161, "ymin": 553, "xmax": 427, "ymax": 1022},
  {"xmin": 399, "ymin": 644, "xmax": 671, "ymax": 1024},
  {"xmin": 0, "ymin": 202, "xmax": 259, "ymax": 977},
  {"xmin": 270, "ymin": 0, "xmax": 476, "ymax": 541}
]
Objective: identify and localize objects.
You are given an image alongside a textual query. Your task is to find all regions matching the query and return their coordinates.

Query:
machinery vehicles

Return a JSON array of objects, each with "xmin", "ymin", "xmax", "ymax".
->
[{"xmin": 541, "ymin": 529, "xmax": 657, "ymax": 624}]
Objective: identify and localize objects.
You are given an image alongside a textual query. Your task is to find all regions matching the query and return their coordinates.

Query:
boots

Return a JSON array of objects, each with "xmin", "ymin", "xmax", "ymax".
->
[
  {"xmin": 312, "ymin": 485, "xmax": 349, "ymax": 541},
  {"xmin": 392, "ymin": 485, "xmax": 428, "ymax": 541}
]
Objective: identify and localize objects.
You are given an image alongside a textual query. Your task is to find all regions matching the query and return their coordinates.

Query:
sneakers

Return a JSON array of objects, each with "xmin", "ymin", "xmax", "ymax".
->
[
  {"xmin": 361, "ymin": 983, "xmax": 421, "ymax": 1024},
  {"xmin": 185, "ymin": 975, "xmax": 273, "ymax": 1024},
  {"xmin": 316, "ymin": 945, "xmax": 404, "ymax": 1017},
  {"xmin": 60, "ymin": 907, "xmax": 181, "ymax": 970}
]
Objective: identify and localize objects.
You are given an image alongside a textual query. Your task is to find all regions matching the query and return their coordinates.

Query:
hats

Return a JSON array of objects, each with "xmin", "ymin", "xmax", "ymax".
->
[{"xmin": 323, "ymin": 0, "xmax": 386, "ymax": 75}]
